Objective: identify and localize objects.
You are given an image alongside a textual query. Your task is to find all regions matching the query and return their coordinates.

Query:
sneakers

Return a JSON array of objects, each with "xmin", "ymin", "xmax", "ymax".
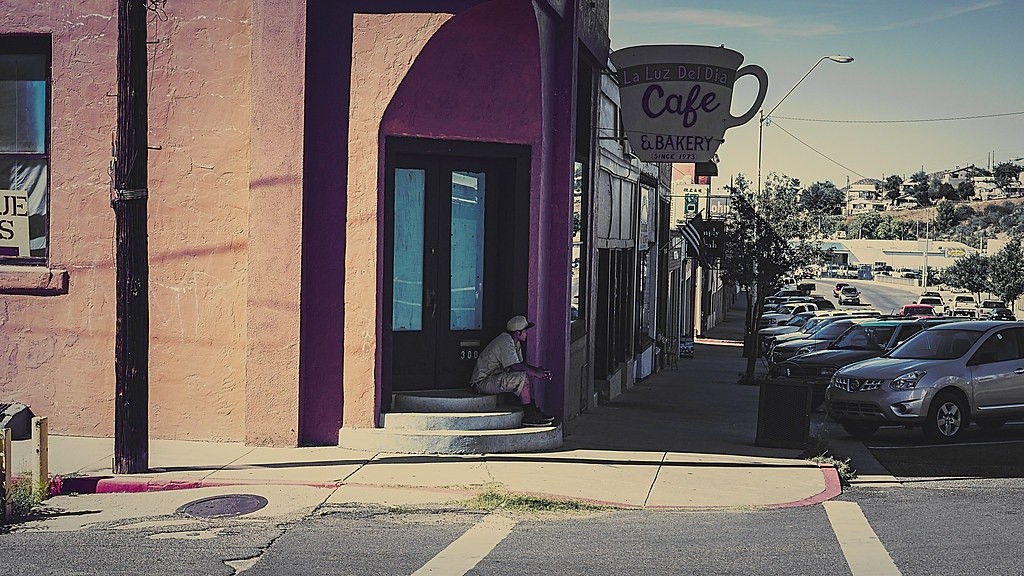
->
[{"xmin": 522, "ymin": 407, "xmax": 554, "ymax": 426}]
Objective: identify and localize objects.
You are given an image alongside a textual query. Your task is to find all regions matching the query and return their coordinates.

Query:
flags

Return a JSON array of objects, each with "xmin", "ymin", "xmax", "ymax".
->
[{"xmin": 682, "ymin": 212, "xmax": 708, "ymax": 274}]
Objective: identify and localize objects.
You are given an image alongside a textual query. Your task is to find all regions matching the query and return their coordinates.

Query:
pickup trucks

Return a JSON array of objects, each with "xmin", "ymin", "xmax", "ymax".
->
[
  {"xmin": 975, "ymin": 300, "xmax": 1005, "ymax": 321},
  {"xmin": 912, "ymin": 297, "xmax": 949, "ymax": 315},
  {"xmin": 900, "ymin": 304, "xmax": 938, "ymax": 319},
  {"xmin": 948, "ymin": 296, "xmax": 978, "ymax": 317}
]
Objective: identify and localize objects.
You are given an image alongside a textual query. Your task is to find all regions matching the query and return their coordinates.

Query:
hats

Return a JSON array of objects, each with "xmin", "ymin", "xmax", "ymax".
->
[{"xmin": 506, "ymin": 315, "xmax": 535, "ymax": 332}]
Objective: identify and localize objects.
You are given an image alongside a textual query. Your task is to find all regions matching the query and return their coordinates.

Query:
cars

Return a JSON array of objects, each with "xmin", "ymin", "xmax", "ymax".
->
[
  {"xmin": 784, "ymin": 322, "xmax": 946, "ymax": 390},
  {"xmin": 758, "ymin": 288, "xmax": 882, "ymax": 362},
  {"xmin": 781, "ymin": 262, "xmax": 917, "ymax": 286},
  {"xmin": 987, "ymin": 308, "xmax": 1016, "ymax": 321},
  {"xmin": 824, "ymin": 322, "xmax": 1024, "ymax": 445},
  {"xmin": 833, "ymin": 283, "xmax": 850, "ymax": 298}
]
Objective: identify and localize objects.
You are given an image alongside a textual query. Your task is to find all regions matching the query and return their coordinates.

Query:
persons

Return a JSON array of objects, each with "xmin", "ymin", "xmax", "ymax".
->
[{"xmin": 469, "ymin": 315, "xmax": 555, "ymax": 426}]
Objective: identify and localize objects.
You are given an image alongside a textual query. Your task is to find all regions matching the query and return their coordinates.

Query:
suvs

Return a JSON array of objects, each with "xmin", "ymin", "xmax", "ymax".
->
[{"xmin": 838, "ymin": 286, "xmax": 861, "ymax": 306}]
